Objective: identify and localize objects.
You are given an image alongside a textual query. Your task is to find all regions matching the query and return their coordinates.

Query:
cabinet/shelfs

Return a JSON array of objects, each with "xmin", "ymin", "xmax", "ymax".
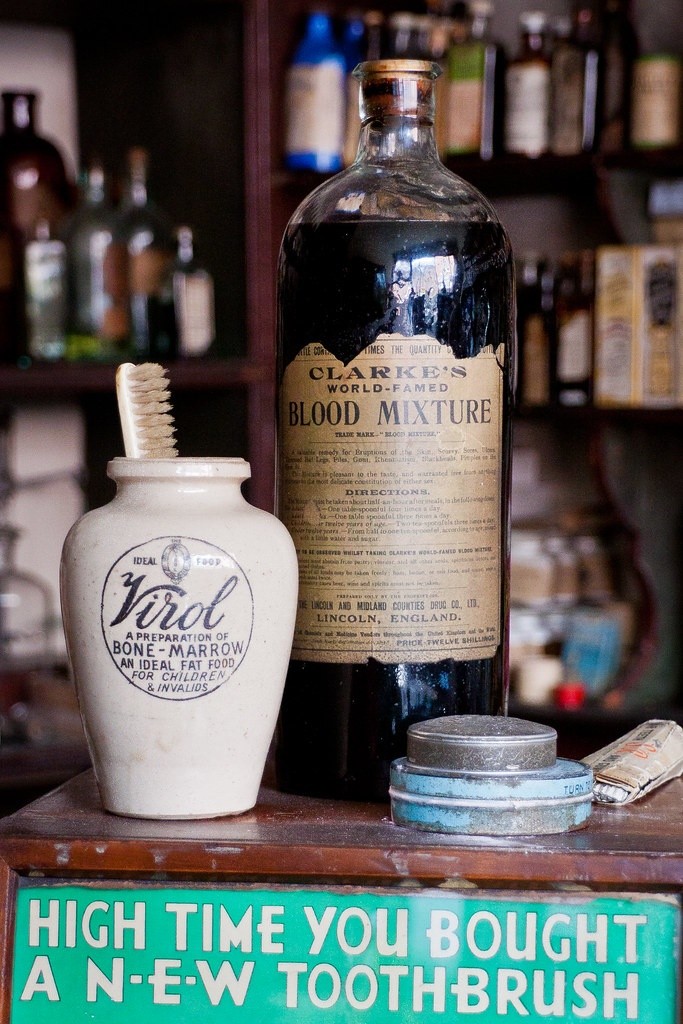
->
[{"xmin": 0, "ymin": 0, "xmax": 683, "ymax": 820}]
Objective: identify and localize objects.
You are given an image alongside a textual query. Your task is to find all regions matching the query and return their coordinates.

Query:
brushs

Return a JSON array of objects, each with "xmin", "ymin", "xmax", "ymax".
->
[{"xmin": 116, "ymin": 361, "xmax": 180, "ymax": 459}]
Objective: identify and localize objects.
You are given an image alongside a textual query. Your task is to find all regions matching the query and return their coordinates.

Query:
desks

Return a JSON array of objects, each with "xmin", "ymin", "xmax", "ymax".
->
[{"xmin": 0, "ymin": 766, "xmax": 683, "ymax": 1024}]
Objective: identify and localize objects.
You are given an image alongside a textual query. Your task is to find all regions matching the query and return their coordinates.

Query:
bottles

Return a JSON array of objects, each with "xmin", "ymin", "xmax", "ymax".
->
[
  {"xmin": 503, "ymin": 12, "xmax": 552, "ymax": 158},
  {"xmin": 278, "ymin": 60, "xmax": 515, "ymax": 799},
  {"xmin": 0, "ymin": 91, "xmax": 69, "ymax": 362},
  {"xmin": 283, "ymin": 8, "xmax": 344, "ymax": 171},
  {"xmin": 447, "ymin": 0, "xmax": 504, "ymax": 161},
  {"xmin": 520, "ymin": 250, "xmax": 596, "ymax": 404},
  {"xmin": 59, "ymin": 459, "xmax": 298, "ymax": 822},
  {"xmin": 72, "ymin": 160, "xmax": 130, "ymax": 354},
  {"xmin": 173, "ymin": 224, "xmax": 215, "ymax": 363},
  {"xmin": 119, "ymin": 151, "xmax": 167, "ymax": 355},
  {"xmin": 553, "ymin": 9, "xmax": 605, "ymax": 154},
  {"xmin": 24, "ymin": 224, "xmax": 67, "ymax": 359}
]
